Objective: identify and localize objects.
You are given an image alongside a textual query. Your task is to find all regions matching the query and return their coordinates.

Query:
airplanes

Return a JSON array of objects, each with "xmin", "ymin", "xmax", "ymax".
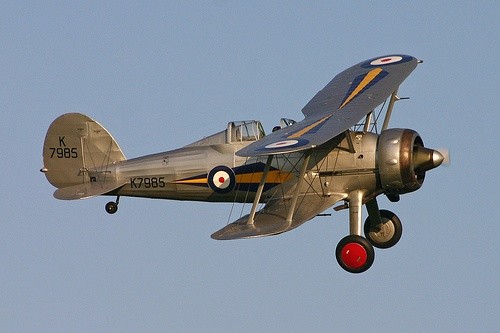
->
[{"xmin": 43, "ymin": 54, "xmax": 448, "ymax": 277}]
